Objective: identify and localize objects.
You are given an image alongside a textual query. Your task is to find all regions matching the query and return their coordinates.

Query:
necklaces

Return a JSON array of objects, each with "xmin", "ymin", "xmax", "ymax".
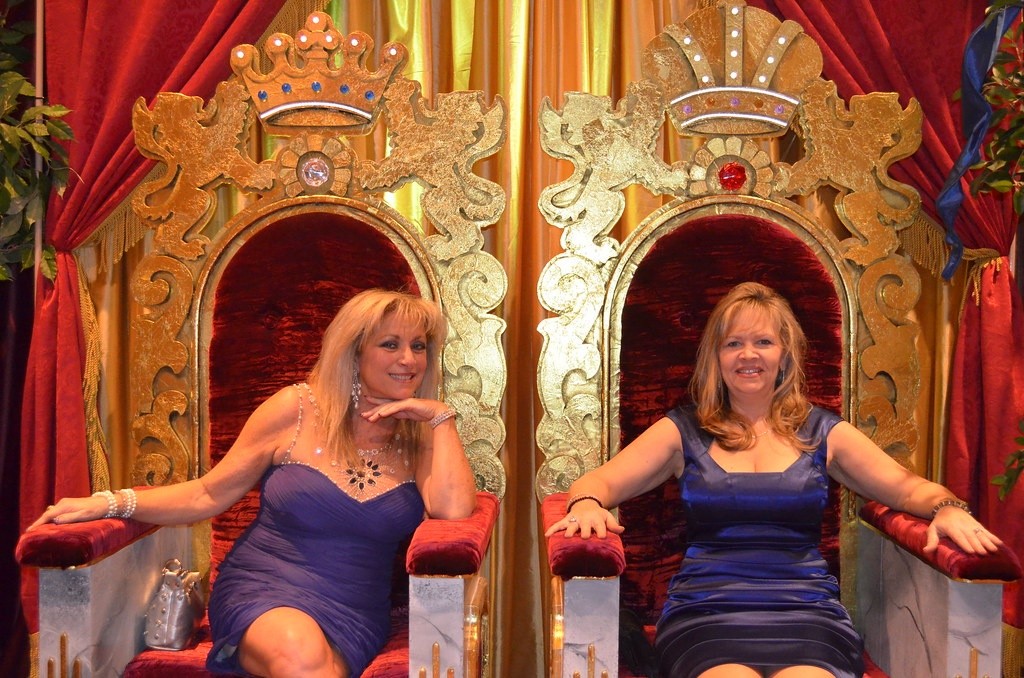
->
[
  {"xmin": 733, "ymin": 418, "xmax": 774, "ymax": 439},
  {"xmin": 303, "ymin": 381, "xmax": 406, "ymax": 457}
]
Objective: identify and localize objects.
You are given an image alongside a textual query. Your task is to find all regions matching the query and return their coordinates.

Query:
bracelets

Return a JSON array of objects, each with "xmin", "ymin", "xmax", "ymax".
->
[
  {"xmin": 113, "ymin": 488, "xmax": 137, "ymax": 519},
  {"xmin": 92, "ymin": 490, "xmax": 117, "ymax": 518},
  {"xmin": 567, "ymin": 492, "xmax": 603, "ymax": 513},
  {"xmin": 931, "ymin": 497, "xmax": 972, "ymax": 518},
  {"xmin": 429, "ymin": 408, "xmax": 459, "ymax": 430}
]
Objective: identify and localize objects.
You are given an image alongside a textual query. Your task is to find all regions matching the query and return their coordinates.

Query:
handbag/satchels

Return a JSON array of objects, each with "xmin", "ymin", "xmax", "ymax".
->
[{"xmin": 145, "ymin": 560, "xmax": 206, "ymax": 652}]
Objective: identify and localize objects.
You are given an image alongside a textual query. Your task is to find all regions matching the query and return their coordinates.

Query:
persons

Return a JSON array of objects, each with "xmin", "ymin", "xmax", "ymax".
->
[
  {"xmin": 24, "ymin": 290, "xmax": 477, "ymax": 678},
  {"xmin": 545, "ymin": 280, "xmax": 1003, "ymax": 678}
]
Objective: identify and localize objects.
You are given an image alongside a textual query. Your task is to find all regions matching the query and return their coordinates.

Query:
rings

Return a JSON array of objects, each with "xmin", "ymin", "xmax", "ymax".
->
[
  {"xmin": 47, "ymin": 505, "xmax": 54, "ymax": 510},
  {"xmin": 974, "ymin": 528, "xmax": 982, "ymax": 533},
  {"xmin": 569, "ymin": 517, "xmax": 576, "ymax": 522}
]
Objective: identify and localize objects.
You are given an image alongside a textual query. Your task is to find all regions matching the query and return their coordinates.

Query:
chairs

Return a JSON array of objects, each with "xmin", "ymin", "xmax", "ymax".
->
[
  {"xmin": 535, "ymin": 4, "xmax": 1024, "ymax": 676},
  {"xmin": 15, "ymin": 7, "xmax": 514, "ymax": 677}
]
